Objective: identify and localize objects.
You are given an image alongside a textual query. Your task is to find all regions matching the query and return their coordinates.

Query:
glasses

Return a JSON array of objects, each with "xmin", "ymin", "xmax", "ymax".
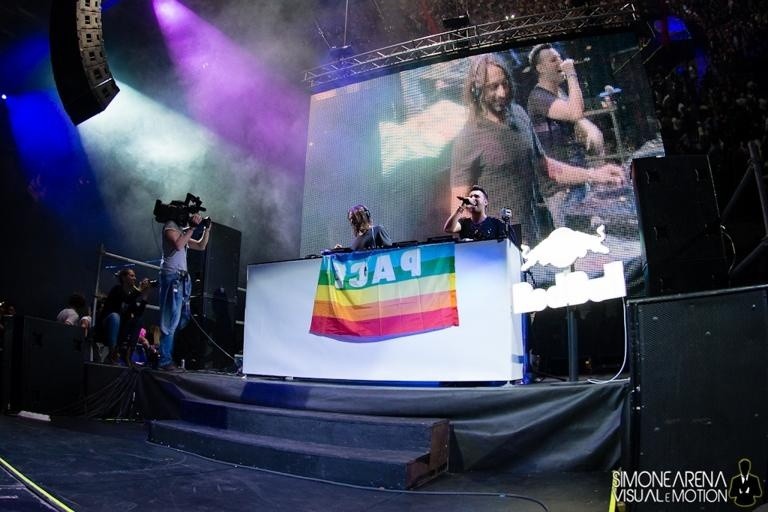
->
[
  {"xmin": 443, "ymin": 15, "xmax": 470, "ymax": 48},
  {"xmin": 329, "ymin": 44, "xmax": 353, "ymax": 76}
]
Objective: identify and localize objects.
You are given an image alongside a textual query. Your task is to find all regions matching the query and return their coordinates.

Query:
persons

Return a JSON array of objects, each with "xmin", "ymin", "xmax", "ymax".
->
[
  {"xmin": 525, "ymin": 42, "xmax": 607, "ymax": 228},
  {"xmin": 334, "ymin": 203, "xmax": 392, "ymax": 248},
  {"xmin": 56, "ymin": 288, "xmax": 86, "ymax": 326},
  {"xmin": 151, "ymin": 200, "xmax": 212, "ymax": 372},
  {"xmin": 442, "ymin": 184, "xmax": 519, "ymax": 246},
  {"xmin": 136, "ymin": 328, "xmax": 160, "ymax": 367},
  {"xmin": 79, "ymin": 301, "xmax": 106, "ymax": 362},
  {"xmin": 448, "ymin": 53, "xmax": 629, "ymax": 248},
  {"xmin": 101, "ymin": 268, "xmax": 148, "ymax": 369},
  {"xmin": 0, "ymin": 302, "xmax": 17, "ymax": 351}
]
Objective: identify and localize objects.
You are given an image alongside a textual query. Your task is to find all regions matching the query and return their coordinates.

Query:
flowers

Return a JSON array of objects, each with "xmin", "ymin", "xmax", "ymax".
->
[
  {"xmin": 574, "ymin": 57, "xmax": 590, "ymax": 67},
  {"xmin": 456, "ymin": 196, "xmax": 477, "ymax": 206}
]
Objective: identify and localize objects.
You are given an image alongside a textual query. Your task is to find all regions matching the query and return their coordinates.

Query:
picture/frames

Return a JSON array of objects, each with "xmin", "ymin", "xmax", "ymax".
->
[{"xmin": 242, "ymin": 238, "xmax": 524, "ymax": 389}]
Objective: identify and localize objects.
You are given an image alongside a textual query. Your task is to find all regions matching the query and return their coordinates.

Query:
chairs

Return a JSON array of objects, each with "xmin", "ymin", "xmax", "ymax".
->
[
  {"xmin": 113, "ymin": 346, "xmax": 121, "ymax": 364},
  {"xmin": 159, "ymin": 362, "xmax": 185, "ymax": 373}
]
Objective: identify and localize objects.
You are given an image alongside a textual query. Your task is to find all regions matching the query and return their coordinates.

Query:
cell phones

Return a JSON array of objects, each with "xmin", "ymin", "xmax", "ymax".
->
[{"xmin": 142, "ymin": 279, "xmax": 159, "ymax": 288}]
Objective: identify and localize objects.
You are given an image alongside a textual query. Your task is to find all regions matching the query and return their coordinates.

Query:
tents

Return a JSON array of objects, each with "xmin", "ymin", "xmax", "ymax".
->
[{"xmin": 470, "ymin": 58, "xmax": 483, "ymax": 97}]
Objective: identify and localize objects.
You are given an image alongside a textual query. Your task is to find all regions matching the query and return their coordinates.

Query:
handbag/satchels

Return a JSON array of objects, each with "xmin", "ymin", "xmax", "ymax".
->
[
  {"xmin": 564, "ymin": 73, "xmax": 577, "ymax": 79},
  {"xmin": 456, "ymin": 206, "xmax": 466, "ymax": 213},
  {"xmin": 205, "ymin": 230, "xmax": 209, "ymax": 233}
]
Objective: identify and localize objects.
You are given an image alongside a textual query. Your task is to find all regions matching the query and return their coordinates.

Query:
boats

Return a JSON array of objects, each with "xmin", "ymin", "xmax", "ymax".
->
[
  {"xmin": 632, "ymin": 154, "xmax": 737, "ymax": 300},
  {"xmin": 624, "ymin": 284, "xmax": 768, "ymax": 512},
  {"xmin": 3, "ymin": 315, "xmax": 84, "ymax": 414},
  {"xmin": 48, "ymin": 0, "xmax": 120, "ymax": 127},
  {"xmin": 184, "ymin": 222, "xmax": 242, "ymax": 369}
]
